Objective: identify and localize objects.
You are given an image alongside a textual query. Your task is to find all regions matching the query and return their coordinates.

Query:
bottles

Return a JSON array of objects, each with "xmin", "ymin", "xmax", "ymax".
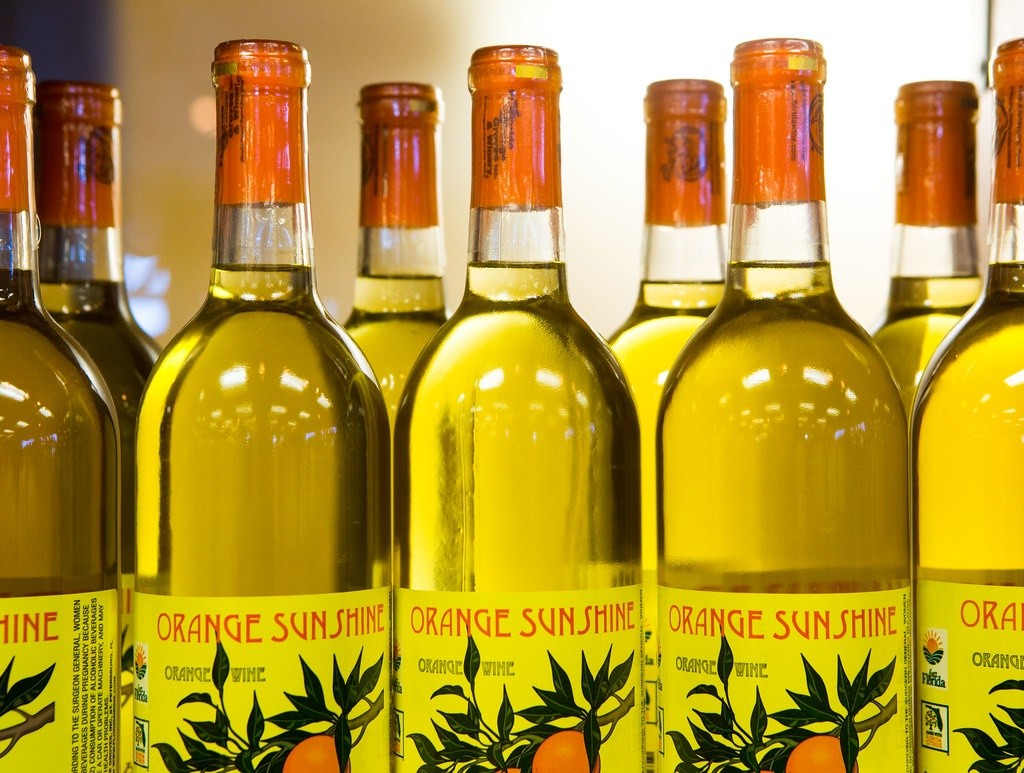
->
[
  {"xmin": 0, "ymin": 44, "xmax": 119, "ymax": 773},
  {"xmin": 341, "ymin": 80, "xmax": 449, "ymax": 428},
  {"xmin": 869, "ymin": 81, "xmax": 985, "ymax": 423},
  {"xmin": 910, "ymin": 36, "xmax": 1024, "ymax": 773},
  {"xmin": 392, "ymin": 43, "xmax": 646, "ymax": 773},
  {"xmin": 36, "ymin": 79, "xmax": 166, "ymax": 773},
  {"xmin": 656, "ymin": 39, "xmax": 914, "ymax": 773},
  {"xmin": 133, "ymin": 39, "xmax": 396, "ymax": 773},
  {"xmin": 606, "ymin": 79, "xmax": 729, "ymax": 773}
]
